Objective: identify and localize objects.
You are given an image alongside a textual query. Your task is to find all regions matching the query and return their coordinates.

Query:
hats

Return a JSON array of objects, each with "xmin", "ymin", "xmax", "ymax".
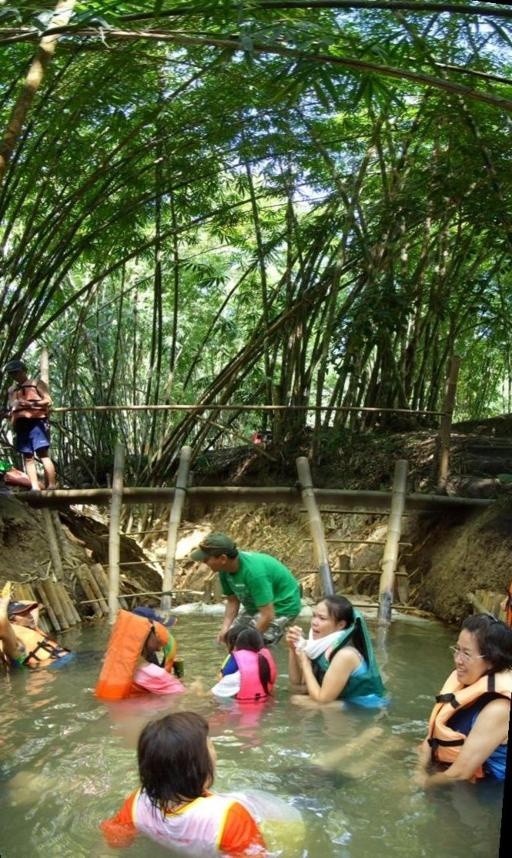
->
[
  {"xmin": 7, "ymin": 361, "xmax": 28, "ymax": 374},
  {"xmin": 132, "ymin": 607, "xmax": 178, "ymax": 626},
  {"xmin": 7, "ymin": 601, "xmax": 39, "ymax": 618},
  {"xmin": 191, "ymin": 533, "xmax": 234, "ymax": 560}
]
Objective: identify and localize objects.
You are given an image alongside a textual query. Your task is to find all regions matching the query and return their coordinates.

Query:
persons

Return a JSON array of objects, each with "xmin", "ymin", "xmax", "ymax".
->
[
  {"xmin": 0, "ymin": 460, "xmax": 46, "ymax": 490},
  {"xmin": 6, "ymin": 359, "xmax": 57, "ymax": 493}
]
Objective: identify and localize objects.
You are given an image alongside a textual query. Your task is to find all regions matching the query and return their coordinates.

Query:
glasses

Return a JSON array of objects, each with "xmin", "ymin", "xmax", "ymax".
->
[{"xmin": 449, "ymin": 645, "xmax": 486, "ymax": 664}]
